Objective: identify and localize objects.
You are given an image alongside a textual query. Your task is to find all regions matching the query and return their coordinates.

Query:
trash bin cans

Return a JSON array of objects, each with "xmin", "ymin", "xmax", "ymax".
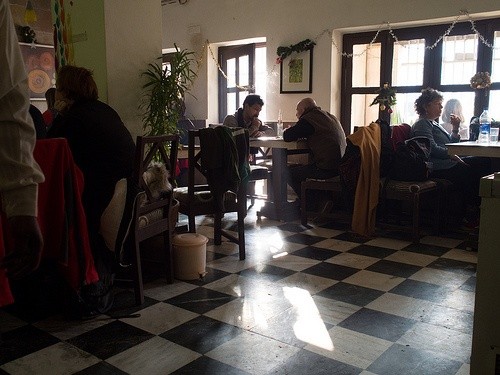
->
[{"xmin": 173, "ymin": 232, "xmax": 208, "ymax": 282}]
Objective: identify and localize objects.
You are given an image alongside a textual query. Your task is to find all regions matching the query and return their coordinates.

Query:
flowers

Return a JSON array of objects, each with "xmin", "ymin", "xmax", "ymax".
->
[
  {"xmin": 470, "ymin": 71, "xmax": 491, "ymax": 96},
  {"xmin": 370, "ymin": 82, "xmax": 396, "ymax": 113}
]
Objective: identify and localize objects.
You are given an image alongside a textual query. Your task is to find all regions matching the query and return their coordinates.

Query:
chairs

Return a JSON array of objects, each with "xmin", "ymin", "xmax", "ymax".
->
[
  {"xmin": 302, "ymin": 125, "xmax": 441, "ymax": 244},
  {"xmin": 0, "ymin": 138, "xmax": 100, "ymax": 321},
  {"xmin": 105, "ymin": 132, "xmax": 179, "ymax": 303},
  {"xmin": 174, "ymin": 125, "xmax": 249, "ymax": 262}
]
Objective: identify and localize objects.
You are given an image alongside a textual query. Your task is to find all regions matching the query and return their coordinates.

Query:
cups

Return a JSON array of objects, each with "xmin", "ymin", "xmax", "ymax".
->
[{"xmin": 490, "ymin": 127, "xmax": 499, "ymax": 143}]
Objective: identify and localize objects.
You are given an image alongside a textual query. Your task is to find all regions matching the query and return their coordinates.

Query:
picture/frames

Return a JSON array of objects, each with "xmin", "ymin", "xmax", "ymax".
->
[
  {"xmin": 18, "ymin": 42, "xmax": 55, "ymax": 101},
  {"xmin": 280, "ymin": 49, "xmax": 313, "ymax": 94}
]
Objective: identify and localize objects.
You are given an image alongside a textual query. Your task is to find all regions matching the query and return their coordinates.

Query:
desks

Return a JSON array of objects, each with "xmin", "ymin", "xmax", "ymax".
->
[
  {"xmin": 444, "ymin": 143, "xmax": 500, "ymax": 254},
  {"xmin": 250, "ymin": 136, "xmax": 314, "ymax": 222}
]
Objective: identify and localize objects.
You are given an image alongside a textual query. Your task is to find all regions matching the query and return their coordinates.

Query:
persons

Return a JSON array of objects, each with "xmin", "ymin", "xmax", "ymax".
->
[
  {"xmin": 410, "ymin": 87, "xmax": 491, "ymax": 228},
  {"xmin": 283, "ymin": 98, "xmax": 347, "ymax": 222},
  {"xmin": 47, "ymin": 66, "xmax": 137, "ymax": 318},
  {"xmin": 43, "ymin": 88, "xmax": 58, "ymax": 128},
  {"xmin": 222, "ymin": 95, "xmax": 275, "ymax": 138},
  {"xmin": 0, "ymin": 0, "xmax": 60, "ymax": 280}
]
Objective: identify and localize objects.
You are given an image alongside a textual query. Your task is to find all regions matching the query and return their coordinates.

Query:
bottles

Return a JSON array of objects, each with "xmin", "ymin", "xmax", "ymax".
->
[
  {"xmin": 277, "ymin": 108, "xmax": 283, "ymax": 137},
  {"xmin": 480, "ymin": 108, "xmax": 491, "ymax": 143}
]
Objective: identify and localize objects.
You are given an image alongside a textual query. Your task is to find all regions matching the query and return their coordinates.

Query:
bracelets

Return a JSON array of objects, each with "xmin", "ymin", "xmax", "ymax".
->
[{"xmin": 452, "ymin": 130, "xmax": 459, "ymax": 134}]
